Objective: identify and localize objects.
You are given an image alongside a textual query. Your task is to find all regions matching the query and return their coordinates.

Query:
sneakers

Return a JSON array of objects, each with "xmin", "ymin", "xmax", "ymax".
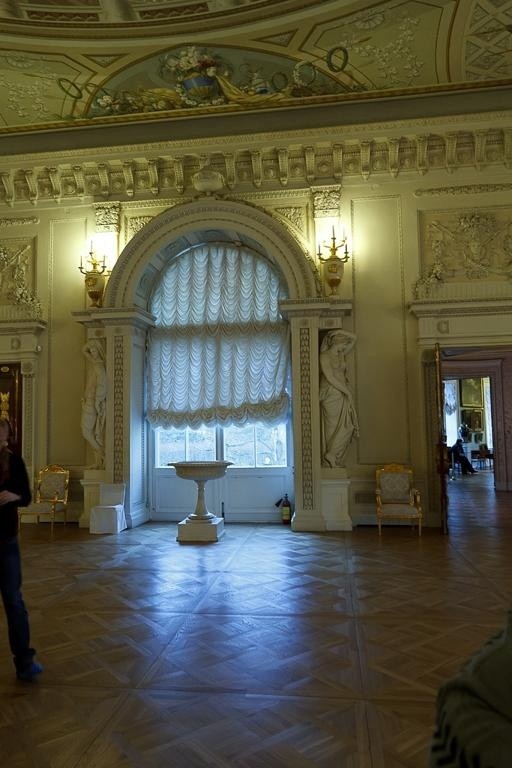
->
[{"xmin": 13, "ymin": 653, "xmax": 43, "ymax": 682}]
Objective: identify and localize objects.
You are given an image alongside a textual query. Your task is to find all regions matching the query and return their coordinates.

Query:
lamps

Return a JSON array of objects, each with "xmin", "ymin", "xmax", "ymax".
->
[
  {"xmin": 317, "ymin": 226, "xmax": 349, "ymax": 297},
  {"xmin": 78, "ymin": 241, "xmax": 111, "ymax": 307}
]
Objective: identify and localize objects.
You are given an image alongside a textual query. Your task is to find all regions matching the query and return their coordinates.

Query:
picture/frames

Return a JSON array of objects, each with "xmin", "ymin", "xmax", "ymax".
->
[{"xmin": 460, "ymin": 377, "xmax": 483, "ymax": 432}]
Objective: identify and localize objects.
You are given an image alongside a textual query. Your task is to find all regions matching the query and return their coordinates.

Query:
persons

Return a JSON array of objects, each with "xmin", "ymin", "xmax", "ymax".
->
[
  {"xmin": 318, "ymin": 326, "xmax": 359, "ymax": 469},
  {"xmin": 0, "ymin": 415, "xmax": 44, "ymax": 682},
  {"xmin": 451, "ymin": 439, "xmax": 479, "ymax": 477},
  {"xmin": 79, "ymin": 338, "xmax": 109, "ymax": 468}
]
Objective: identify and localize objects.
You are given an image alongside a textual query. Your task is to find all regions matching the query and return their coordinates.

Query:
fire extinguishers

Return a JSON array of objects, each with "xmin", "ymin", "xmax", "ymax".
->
[{"xmin": 275, "ymin": 493, "xmax": 291, "ymax": 525}]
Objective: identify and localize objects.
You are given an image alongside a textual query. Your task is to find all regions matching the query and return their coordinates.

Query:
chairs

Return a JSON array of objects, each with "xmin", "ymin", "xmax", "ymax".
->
[
  {"xmin": 17, "ymin": 464, "xmax": 70, "ymax": 537},
  {"xmin": 452, "ymin": 444, "xmax": 493, "ymax": 475},
  {"xmin": 90, "ymin": 483, "xmax": 128, "ymax": 535},
  {"xmin": 375, "ymin": 463, "xmax": 423, "ymax": 536}
]
